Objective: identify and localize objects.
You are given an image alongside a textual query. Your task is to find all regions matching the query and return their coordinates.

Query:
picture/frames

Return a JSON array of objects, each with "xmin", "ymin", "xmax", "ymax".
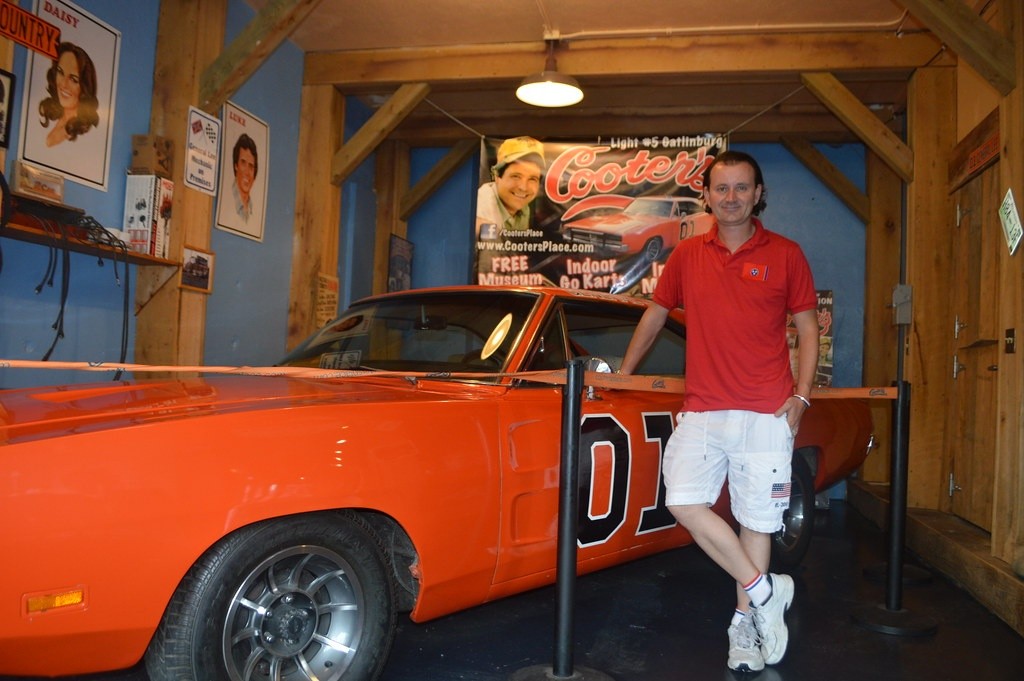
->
[
  {"xmin": 10, "ymin": 161, "xmax": 65, "ymax": 203},
  {"xmin": 177, "ymin": 241, "xmax": 217, "ymax": 293},
  {"xmin": 386, "ymin": 232, "xmax": 415, "ymax": 292},
  {"xmin": 0, "ymin": 68, "xmax": 17, "ymax": 148}
]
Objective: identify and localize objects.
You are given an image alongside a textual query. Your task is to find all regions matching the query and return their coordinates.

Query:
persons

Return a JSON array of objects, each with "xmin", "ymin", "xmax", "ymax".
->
[
  {"xmin": 474, "ymin": 135, "xmax": 548, "ymax": 286},
  {"xmin": 606, "ymin": 150, "xmax": 819, "ymax": 672}
]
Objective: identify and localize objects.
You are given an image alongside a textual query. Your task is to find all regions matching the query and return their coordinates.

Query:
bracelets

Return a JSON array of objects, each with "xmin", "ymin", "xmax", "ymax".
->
[
  {"xmin": 614, "ymin": 370, "xmax": 624, "ymax": 374},
  {"xmin": 793, "ymin": 394, "xmax": 810, "ymax": 408}
]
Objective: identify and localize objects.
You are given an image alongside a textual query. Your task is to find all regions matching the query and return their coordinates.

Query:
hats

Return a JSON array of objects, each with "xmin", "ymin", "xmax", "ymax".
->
[
  {"xmin": 497, "ymin": 136, "xmax": 546, "ymax": 169},
  {"xmin": 820, "ymin": 338, "xmax": 830, "ymax": 345}
]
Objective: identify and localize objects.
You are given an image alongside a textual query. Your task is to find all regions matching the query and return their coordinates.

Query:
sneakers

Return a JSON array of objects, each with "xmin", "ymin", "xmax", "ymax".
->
[
  {"xmin": 727, "ymin": 614, "xmax": 765, "ymax": 672},
  {"xmin": 748, "ymin": 573, "xmax": 794, "ymax": 665}
]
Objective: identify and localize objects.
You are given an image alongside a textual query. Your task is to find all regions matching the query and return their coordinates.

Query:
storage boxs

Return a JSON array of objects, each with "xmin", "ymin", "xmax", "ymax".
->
[
  {"xmin": 131, "ymin": 133, "xmax": 174, "ymax": 180},
  {"xmin": 122, "ymin": 174, "xmax": 175, "ymax": 260}
]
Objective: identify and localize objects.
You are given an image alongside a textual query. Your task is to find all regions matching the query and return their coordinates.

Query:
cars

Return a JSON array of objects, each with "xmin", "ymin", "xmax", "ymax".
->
[
  {"xmin": 560, "ymin": 197, "xmax": 716, "ymax": 261},
  {"xmin": 1, "ymin": 286, "xmax": 877, "ymax": 681}
]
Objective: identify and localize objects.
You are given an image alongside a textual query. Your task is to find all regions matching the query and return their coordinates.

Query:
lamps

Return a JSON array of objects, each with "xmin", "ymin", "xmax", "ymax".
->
[{"xmin": 515, "ymin": 29, "xmax": 584, "ymax": 106}]
